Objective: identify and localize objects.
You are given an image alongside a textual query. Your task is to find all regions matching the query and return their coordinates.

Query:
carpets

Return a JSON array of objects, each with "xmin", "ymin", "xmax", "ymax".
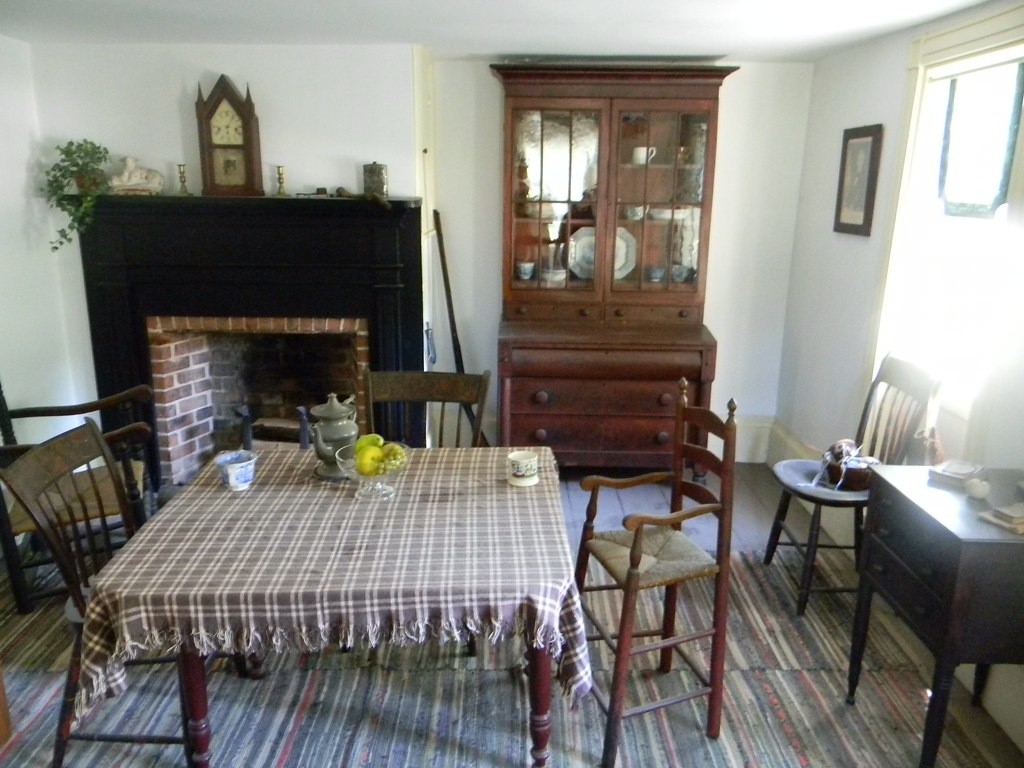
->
[{"xmin": 0, "ymin": 547, "xmax": 1023, "ymax": 768}]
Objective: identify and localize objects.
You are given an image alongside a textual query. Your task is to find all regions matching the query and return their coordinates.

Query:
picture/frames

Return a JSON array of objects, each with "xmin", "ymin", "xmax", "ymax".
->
[{"xmin": 832, "ymin": 122, "xmax": 886, "ymax": 243}]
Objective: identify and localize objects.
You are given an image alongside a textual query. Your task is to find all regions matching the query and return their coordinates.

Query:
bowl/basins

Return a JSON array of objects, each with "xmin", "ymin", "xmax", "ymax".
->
[{"xmin": 649, "ymin": 205, "xmax": 693, "ymax": 220}]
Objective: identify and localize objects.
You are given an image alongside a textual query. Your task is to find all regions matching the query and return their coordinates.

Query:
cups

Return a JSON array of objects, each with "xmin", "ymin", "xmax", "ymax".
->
[
  {"xmin": 647, "ymin": 267, "xmax": 666, "ymax": 281},
  {"xmin": 214, "ymin": 450, "xmax": 258, "ymax": 491},
  {"xmin": 516, "ymin": 262, "xmax": 535, "ymax": 279},
  {"xmin": 508, "ymin": 451, "xmax": 540, "ymax": 487},
  {"xmin": 668, "ymin": 145, "xmax": 685, "ymax": 165},
  {"xmin": 623, "ymin": 204, "xmax": 649, "ymax": 220},
  {"xmin": 673, "ymin": 265, "xmax": 693, "ymax": 282},
  {"xmin": 632, "ymin": 146, "xmax": 656, "ymax": 165}
]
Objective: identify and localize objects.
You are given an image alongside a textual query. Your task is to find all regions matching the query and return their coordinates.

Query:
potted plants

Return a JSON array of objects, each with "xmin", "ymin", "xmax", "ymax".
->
[{"xmin": 39, "ymin": 136, "xmax": 114, "ymax": 252}]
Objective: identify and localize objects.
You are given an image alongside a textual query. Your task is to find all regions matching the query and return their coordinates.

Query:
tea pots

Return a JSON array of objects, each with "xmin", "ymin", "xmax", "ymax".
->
[{"xmin": 309, "ymin": 392, "xmax": 359, "ymax": 479}]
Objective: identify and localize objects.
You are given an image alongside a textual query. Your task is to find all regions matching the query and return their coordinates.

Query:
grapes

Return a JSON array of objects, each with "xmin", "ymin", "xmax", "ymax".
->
[{"xmin": 377, "ymin": 449, "xmax": 406, "ymax": 475}]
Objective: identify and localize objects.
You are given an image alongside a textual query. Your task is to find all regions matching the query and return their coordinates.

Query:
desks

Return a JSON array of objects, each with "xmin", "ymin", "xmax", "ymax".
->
[
  {"xmin": 844, "ymin": 465, "xmax": 1024, "ymax": 768},
  {"xmin": 73, "ymin": 443, "xmax": 594, "ymax": 768}
]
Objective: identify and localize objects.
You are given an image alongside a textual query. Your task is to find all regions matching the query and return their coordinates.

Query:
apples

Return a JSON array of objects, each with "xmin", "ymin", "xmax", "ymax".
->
[
  {"xmin": 381, "ymin": 443, "xmax": 401, "ymax": 455},
  {"xmin": 355, "ymin": 446, "xmax": 385, "ymax": 475}
]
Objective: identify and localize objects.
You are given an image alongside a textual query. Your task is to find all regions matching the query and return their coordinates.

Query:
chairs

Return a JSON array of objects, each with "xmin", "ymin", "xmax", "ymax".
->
[
  {"xmin": 0, "ymin": 378, "xmax": 186, "ymax": 768},
  {"xmin": 763, "ymin": 357, "xmax": 936, "ymax": 616},
  {"xmin": 361, "ymin": 363, "xmax": 479, "ymax": 658},
  {"xmin": 575, "ymin": 374, "xmax": 739, "ymax": 768}
]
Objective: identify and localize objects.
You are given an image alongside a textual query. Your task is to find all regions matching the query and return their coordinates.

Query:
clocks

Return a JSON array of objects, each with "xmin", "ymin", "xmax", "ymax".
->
[{"xmin": 195, "ymin": 72, "xmax": 265, "ymax": 199}]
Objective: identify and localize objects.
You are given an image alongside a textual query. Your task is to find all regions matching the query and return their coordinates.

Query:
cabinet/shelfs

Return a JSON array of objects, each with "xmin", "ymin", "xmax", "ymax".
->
[{"xmin": 487, "ymin": 60, "xmax": 741, "ymax": 488}]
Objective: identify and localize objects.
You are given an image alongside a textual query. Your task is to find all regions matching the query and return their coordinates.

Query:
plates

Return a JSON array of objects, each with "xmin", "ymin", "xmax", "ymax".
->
[{"xmin": 568, "ymin": 226, "xmax": 635, "ymax": 280}]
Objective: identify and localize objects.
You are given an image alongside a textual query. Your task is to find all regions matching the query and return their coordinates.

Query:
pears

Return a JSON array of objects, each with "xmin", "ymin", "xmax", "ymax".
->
[{"xmin": 355, "ymin": 433, "xmax": 384, "ymax": 454}]
